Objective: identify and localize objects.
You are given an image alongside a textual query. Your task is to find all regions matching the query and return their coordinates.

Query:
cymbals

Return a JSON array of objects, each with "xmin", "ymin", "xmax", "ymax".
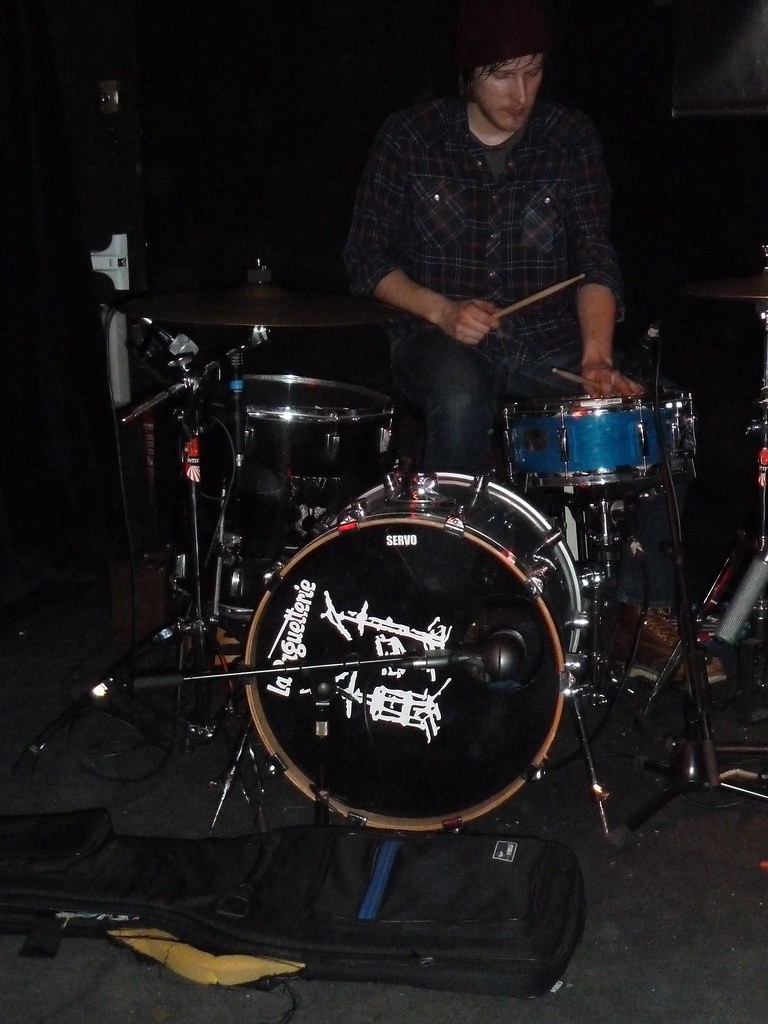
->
[
  {"xmin": 686, "ymin": 268, "xmax": 768, "ymax": 301},
  {"xmin": 115, "ymin": 285, "xmax": 399, "ymax": 332}
]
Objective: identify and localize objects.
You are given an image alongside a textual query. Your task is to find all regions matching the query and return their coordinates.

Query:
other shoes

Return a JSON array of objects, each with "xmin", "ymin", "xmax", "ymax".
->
[{"xmin": 614, "ymin": 605, "xmax": 726, "ymax": 685}]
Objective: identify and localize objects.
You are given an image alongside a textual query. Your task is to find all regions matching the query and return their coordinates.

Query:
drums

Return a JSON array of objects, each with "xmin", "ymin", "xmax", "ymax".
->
[
  {"xmin": 189, "ymin": 371, "xmax": 397, "ymax": 503},
  {"xmin": 496, "ymin": 389, "xmax": 702, "ymax": 497},
  {"xmin": 238, "ymin": 466, "xmax": 590, "ymax": 839}
]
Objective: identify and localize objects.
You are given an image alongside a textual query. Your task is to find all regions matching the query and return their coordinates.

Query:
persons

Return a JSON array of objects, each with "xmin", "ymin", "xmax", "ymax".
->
[{"xmin": 344, "ymin": 0, "xmax": 729, "ymax": 689}]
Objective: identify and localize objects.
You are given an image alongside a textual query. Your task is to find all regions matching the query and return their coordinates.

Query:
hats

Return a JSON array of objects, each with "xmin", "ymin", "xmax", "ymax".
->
[{"xmin": 456, "ymin": 0, "xmax": 547, "ymax": 66}]
